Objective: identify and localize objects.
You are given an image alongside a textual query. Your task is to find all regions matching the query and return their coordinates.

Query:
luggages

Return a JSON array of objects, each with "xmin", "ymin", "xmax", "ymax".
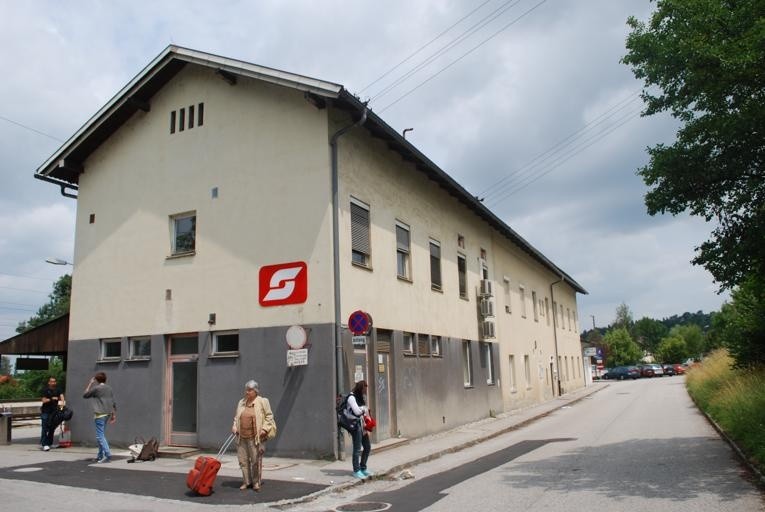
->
[{"xmin": 185, "ymin": 432, "xmax": 237, "ymax": 496}]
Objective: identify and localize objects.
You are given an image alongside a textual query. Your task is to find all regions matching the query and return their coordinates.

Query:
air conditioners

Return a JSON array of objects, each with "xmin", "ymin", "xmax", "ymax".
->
[
  {"xmin": 482, "ymin": 320, "xmax": 496, "ymax": 338},
  {"xmin": 479, "ymin": 278, "xmax": 494, "ymax": 296},
  {"xmin": 480, "ymin": 299, "xmax": 495, "ymax": 316}
]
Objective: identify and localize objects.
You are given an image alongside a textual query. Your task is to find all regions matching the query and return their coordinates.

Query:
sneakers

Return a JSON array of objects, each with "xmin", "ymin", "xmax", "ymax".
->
[
  {"xmin": 40, "ymin": 443, "xmax": 57, "ymax": 453},
  {"xmin": 239, "ymin": 483, "xmax": 262, "ymax": 492},
  {"xmin": 351, "ymin": 469, "xmax": 373, "ymax": 480},
  {"xmin": 92, "ymin": 455, "xmax": 111, "ymax": 466}
]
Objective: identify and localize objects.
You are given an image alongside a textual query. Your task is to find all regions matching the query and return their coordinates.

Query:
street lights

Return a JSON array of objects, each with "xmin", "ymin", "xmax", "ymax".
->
[{"xmin": 704, "ymin": 322, "xmax": 711, "ymax": 359}]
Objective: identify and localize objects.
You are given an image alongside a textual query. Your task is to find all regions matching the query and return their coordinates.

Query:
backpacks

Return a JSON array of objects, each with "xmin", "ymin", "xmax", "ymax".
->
[{"xmin": 335, "ymin": 392, "xmax": 358, "ymax": 414}]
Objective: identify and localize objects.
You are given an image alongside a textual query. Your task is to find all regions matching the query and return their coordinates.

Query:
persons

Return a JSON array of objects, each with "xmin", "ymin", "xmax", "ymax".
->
[
  {"xmin": 40, "ymin": 376, "xmax": 65, "ymax": 451},
  {"xmin": 231, "ymin": 380, "xmax": 277, "ymax": 491},
  {"xmin": 82, "ymin": 372, "xmax": 117, "ymax": 463},
  {"xmin": 336, "ymin": 380, "xmax": 376, "ymax": 479}
]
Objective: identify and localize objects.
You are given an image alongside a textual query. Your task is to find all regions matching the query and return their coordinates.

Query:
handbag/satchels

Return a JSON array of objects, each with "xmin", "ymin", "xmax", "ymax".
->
[
  {"xmin": 262, "ymin": 416, "xmax": 277, "ymax": 439},
  {"xmin": 128, "ymin": 434, "xmax": 160, "ymax": 463},
  {"xmin": 362, "ymin": 415, "xmax": 375, "ymax": 432},
  {"xmin": 338, "ymin": 410, "xmax": 359, "ymax": 433},
  {"xmin": 59, "ymin": 407, "xmax": 72, "ymax": 422}
]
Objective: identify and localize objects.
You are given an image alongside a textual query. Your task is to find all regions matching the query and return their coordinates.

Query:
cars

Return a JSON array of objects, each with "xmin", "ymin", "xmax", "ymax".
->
[{"xmin": 590, "ymin": 361, "xmax": 693, "ymax": 380}]
